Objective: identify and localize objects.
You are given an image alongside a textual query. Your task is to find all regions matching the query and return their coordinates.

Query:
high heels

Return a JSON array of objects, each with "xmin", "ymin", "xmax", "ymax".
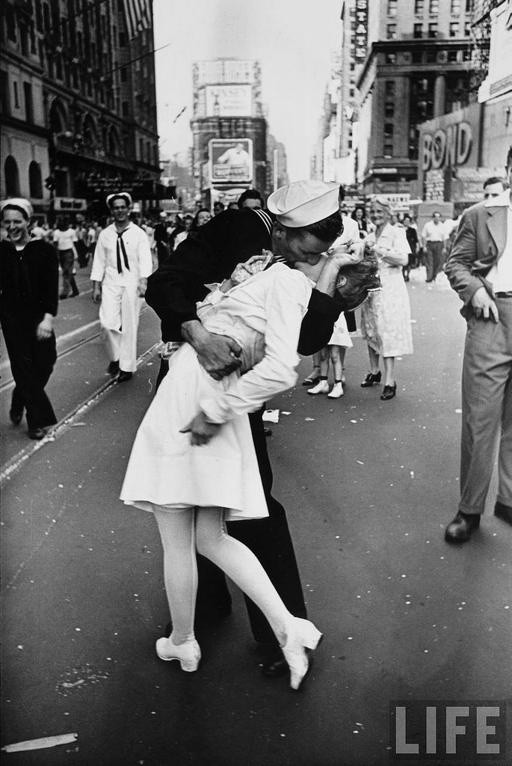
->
[
  {"xmin": 361, "ymin": 371, "xmax": 381, "ymax": 387},
  {"xmin": 155, "ymin": 638, "xmax": 202, "ymax": 673},
  {"xmin": 281, "ymin": 618, "xmax": 324, "ymax": 690},
  {"xmin": 380, "ymin": 382, "xmax": 396, "ymax": 400}
]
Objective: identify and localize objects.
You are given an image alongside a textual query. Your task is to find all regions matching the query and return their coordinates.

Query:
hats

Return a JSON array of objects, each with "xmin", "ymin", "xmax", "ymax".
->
[
  {"xmin": 267, "ymin": 181, "xmax": 340, "ymax": 228},
  {"xmin": 0, "ymin": 198, "xmax": 34, "ymax": 220},
  {"xmin": 106, "ymin": 192, "xmax": 132, "ymax": 208}
]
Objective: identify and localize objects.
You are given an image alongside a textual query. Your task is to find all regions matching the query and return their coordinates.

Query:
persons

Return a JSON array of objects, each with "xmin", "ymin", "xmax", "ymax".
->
[
  {"xmin": 218, "ymin": 142, "xmax": 250, "ymax": 177},
  {"xmin": 441, "ymin": 136, "xmax": 512, "ymax": 545},
  {"xmin": 141, "ymin": 176, "xmax": 346, "ymax": 682},
  {"xmin": 112, "ymin": 236, "xmax": 383, "ymax": 695},
  {"xmin": 1, "ymin": 176, "xmax": 511, "ymax": 444}
]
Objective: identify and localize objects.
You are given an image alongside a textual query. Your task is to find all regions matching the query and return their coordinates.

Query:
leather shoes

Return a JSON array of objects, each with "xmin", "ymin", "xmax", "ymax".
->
[
  {"xmin": 10, "ymin": 387, "xmax": 25, "ymax": 426},
  {"xmin": 446, "ymin": 510, "xmax": 480, "ymax": 543},
  {"xmin": 302, "ymin": 365, "xmax": 346, "ymax": 399},
  {"xmin": 108, "ymin": 361, "xmax": 131, "ymax": 381},
  {"xmin": 28, "ymin": 428, "xmax": 47, "ymax": 440}
]
